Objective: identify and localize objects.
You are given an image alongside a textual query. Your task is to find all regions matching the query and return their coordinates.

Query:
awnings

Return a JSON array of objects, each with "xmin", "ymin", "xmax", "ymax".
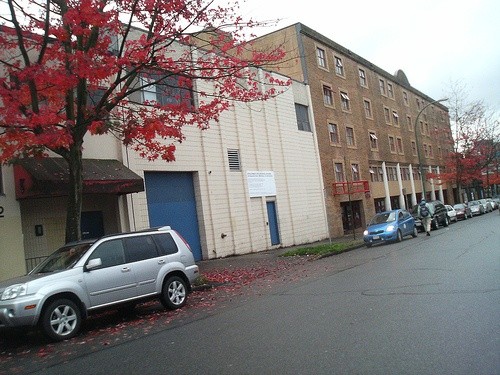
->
[{"xmin": 13, "ymin": 157, "xmax": 144, "ymax": 201}]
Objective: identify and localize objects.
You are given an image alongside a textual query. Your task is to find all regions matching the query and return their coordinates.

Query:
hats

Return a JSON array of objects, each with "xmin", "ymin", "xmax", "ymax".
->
[{"xmin": 421, "ymin": 198, "xmax": 425, "ymax": 200}]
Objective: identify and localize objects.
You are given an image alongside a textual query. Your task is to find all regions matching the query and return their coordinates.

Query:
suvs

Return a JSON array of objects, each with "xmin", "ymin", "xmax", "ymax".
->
[
  {"xmin": 0, "ymin": 226, "xmax": 199, "ymax": 342},
  {"xmin": 411, "ymin": 200, "xmax": 449, "ymax": 233}
]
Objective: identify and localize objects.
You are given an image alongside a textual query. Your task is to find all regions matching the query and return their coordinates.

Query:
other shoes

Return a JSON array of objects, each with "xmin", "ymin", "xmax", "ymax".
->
[{"xmin": 426, "ymin": 231, "xmax": 430, "ymax": 237}]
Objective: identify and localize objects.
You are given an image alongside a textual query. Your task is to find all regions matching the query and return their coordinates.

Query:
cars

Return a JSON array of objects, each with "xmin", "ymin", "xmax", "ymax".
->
[
  {"xmin": 363, "ymin": 209, "xmax": 418, "ymax": 248},
  {"xmin": 444, "ymin": 198, "xmax": 500, "ymax": 223}
]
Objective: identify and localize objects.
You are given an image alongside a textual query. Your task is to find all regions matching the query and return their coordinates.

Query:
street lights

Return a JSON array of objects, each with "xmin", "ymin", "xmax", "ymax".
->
[{"xmin": 414, "ymin": 98, "xmax": 450, "ymax": 199}]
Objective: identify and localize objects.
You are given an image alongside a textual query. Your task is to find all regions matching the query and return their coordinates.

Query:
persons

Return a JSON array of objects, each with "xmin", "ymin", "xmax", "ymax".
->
[{"xmin": 417, "ymin": 198, "xmax": 433, "ymax": 236}]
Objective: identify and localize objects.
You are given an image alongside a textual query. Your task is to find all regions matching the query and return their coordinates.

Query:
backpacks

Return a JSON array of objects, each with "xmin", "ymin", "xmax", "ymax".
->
[{"xmin": 419, "ymin": 202, "xmax": 429, "ymax": 217}]
